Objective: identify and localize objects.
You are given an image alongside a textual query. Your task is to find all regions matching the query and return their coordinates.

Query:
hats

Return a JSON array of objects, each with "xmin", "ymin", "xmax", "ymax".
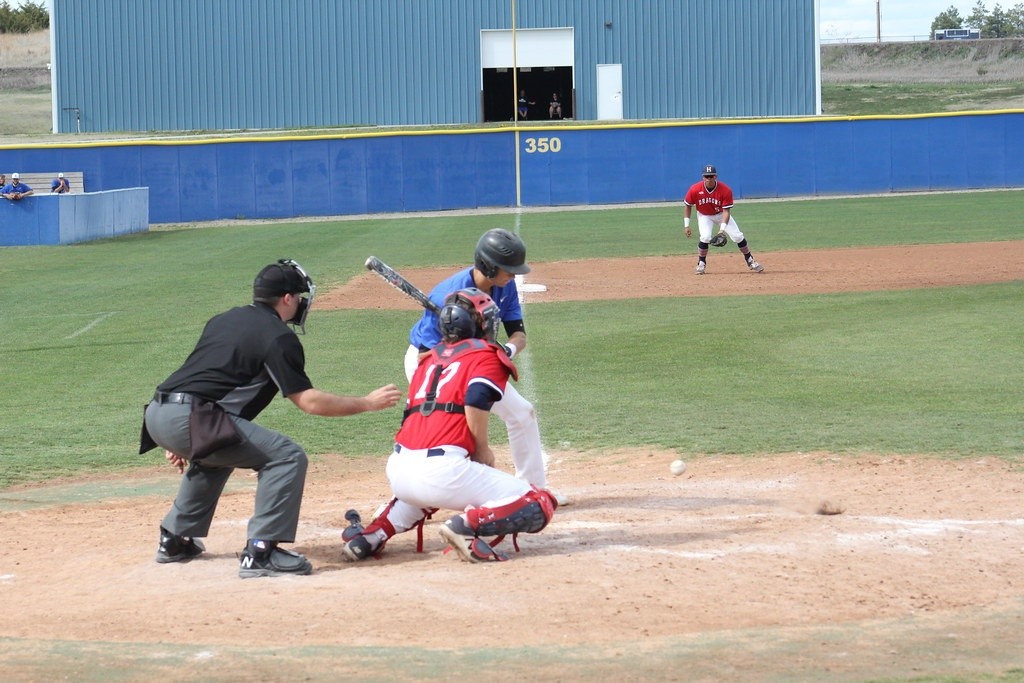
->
[
  {"xmin": 57, "ymin": 172, "xmax": 64, "ymax": 178},
  {"xmin": 254, "ymin": 263, "xmax": 310, "ymax": 298},
  {"xmin": 11, "ymin": 173, "xmax": 20, "ymax": 180}
]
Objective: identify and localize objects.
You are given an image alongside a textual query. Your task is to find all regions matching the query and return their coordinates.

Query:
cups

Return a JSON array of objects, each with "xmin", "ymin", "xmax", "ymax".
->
[{"xmin": 15, "ymin": 192, "xmax": 19, "ymax": 199}]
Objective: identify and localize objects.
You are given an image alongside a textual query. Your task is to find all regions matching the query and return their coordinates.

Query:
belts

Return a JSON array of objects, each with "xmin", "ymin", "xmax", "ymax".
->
[
  {"xmin": 154, "ymin": 391, "xmax": 194, "ymax": 403},
  {"xmin": 394, "ymin": 444, "xmax": 444, "ymax": 457}
]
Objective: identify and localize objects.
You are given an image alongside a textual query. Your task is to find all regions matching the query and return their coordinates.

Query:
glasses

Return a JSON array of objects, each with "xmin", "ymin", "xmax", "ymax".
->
[{"xmin": 704, "ymin": 175, "xmax": 715, "ymax": 179}]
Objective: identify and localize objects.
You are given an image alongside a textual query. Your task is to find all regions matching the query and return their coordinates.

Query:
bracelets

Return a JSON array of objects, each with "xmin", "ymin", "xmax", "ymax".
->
[
  {"xmin": 684, "ymin": 218, "xmax": 690, "ymax": 227},
  {"xmin": 720, "ymin": 223, "xmax": 727, "ymax": 230}
]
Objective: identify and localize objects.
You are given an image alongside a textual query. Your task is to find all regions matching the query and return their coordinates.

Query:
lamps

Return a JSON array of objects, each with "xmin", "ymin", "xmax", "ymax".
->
[{"xmin": 605, "ymin": 21, "xmax": 611, "ymax": 26}]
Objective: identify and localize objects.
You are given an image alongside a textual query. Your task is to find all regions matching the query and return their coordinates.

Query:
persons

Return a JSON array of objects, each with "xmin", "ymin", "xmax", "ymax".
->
[
  {"xmin": 135, "ymin": 259, "xmax": 403, "ymax": 578},
  {"xmin": 51, "ymin": 173, "xmax": 70, "ymax": 194},
  {"xmin": 340, "ymin": 286, "xmax": 559, "ymax": 564},
  {"xmin": 404, "ymin": 226, "xmax": 569, "ymax": 507},
  {"xmin": 684, "ymin": 165, "xmax": 766, "ymax": 274},
  {"xmin": 549, "ymin": 92, "xmax": 563, "ymax": 120},
  {"xmin": 518, "ymin": 89, "xmax": 537, "ymax": 120},
  {"xmin": 0, "ymin": 173, "xmax": 34, "ymax": 199}
]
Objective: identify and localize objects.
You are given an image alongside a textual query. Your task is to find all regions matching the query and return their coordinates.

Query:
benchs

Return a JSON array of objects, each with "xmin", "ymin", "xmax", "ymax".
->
[{"xmin": 0, "ymin": 172, "xmax": 84, "ymax": 195}]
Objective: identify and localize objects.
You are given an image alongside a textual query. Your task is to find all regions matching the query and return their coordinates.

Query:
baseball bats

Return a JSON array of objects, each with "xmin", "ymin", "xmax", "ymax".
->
[{"xmin": 364, "ymin": 255, "xmax": 441, "ymax": 314}]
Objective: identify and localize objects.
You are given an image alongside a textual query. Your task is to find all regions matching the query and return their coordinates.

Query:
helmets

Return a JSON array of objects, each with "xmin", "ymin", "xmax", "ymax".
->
[
  {"xmin": 439, "ymin": 287, "xmax": 497, "ymax": 339},
  {"xmin": 702, "ymin": 165, "xmax": 717, "ymax": 175},
  {"xmin": 474, "ymin": 228, "xmax": 530, "ymax": 279}
]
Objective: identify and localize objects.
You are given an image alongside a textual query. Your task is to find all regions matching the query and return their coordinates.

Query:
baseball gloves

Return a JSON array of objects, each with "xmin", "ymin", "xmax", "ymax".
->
[{"xmin": 710, "ymin": 233, "xmax": 728, "ymax": 247}]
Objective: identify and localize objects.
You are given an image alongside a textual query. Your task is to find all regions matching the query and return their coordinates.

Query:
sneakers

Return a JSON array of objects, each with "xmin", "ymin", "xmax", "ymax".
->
[
  {"xmin": 156, "ymin": 533, "xmax": 204, "ymax": 563},
  {"xmin": 440, "ymin": 513, "xmax": 489, "ymax": 563},
  {"xmin": 695, "ymin": 261, "xmax": 706, "ymax": 275},
  {"xmin": 747, "ymin": 257, "xmax": 764, "ymax": 272},
  {"xmin": 238, "ymin": 544, "xmax": 312, "ymax": 579},
  {"xmin": 344, "ymin": 533, "xmax": 375, "ymax": 563}
]
